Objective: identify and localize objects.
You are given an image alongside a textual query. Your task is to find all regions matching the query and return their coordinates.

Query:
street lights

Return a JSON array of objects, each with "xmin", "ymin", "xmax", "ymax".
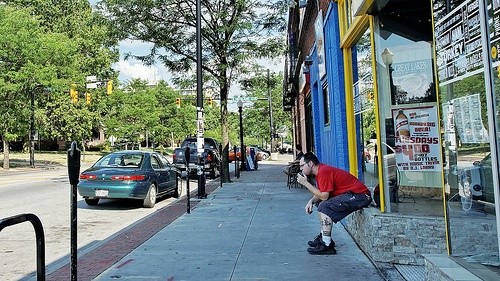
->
[{"xmin": 236, "ymin": 100, "xmax": 245, "ymax": 170}]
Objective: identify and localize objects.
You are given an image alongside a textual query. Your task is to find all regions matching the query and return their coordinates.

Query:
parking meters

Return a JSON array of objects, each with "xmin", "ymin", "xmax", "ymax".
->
[
  {"xmin": 219, "ymin": 144, "xmax": 223, "ymax": 188},
  {"xmin": 184, "ymin": 144, "xmax": 191, "ymax": 214},
  {"xmin": 67, "ymin": 141, "xmax": 81, "ymax": 281}
]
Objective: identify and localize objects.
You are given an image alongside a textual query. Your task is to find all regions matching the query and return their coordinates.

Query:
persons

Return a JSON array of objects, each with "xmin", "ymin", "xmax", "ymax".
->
[
  {"xmin": 283, "ymin": 145, "xmax": 305, "ymax": 183},
  {"xmin": 297, "ymin": 152, "xmax": 372, "ymax": 255}
]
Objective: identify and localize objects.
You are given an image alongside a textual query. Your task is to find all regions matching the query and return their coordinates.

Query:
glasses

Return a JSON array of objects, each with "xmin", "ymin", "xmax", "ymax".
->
[{"xmin": 299, "ymin": 163, "xmax": 306, "ymax": 167}]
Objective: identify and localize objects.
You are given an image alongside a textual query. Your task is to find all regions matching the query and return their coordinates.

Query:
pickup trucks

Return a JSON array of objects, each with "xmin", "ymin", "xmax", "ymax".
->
[{"xmin": 173, "ymin": 137, "xmax": 219, "ymax": 179}]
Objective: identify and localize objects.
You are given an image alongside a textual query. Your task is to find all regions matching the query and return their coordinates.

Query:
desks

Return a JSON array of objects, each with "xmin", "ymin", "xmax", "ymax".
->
[{"xmin": 282, "ymin": 166, "xmax": 302, "ymax": 187}]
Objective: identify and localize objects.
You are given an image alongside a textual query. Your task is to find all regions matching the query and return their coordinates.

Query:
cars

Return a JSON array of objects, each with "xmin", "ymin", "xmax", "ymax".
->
[
  {"xmin": 460, "ymin": 152, "xmax": 495, "ymax": 204},
  {"xmin": 228, "ymin": 145, "xmax": 270, "ymax": 163},
  {"xmin": 365, "ymin": 143, "xmax": 396, "ymax": 181},
  {"xmin": 78, "ymin": 150, "xmax": 182, "ymax": 208}
]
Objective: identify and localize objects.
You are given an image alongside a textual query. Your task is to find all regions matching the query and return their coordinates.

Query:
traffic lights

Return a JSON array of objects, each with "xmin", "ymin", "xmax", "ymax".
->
[
  {"xmin": 85, "ymin": 91, "xmax": 91, "ymax": 105},
  {"xmin": 72, "ymin": 91, "xmax": 78, "ymax": 102},
  {"xmin": 175, "ymin": 98, "xmax": 180, "ymax": 109},
  {"xmin": 207, "ymin": 98, "xmax": 212, "ymax": 110}
]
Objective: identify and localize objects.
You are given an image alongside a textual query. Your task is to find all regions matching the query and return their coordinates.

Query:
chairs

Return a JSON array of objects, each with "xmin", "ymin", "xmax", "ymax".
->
[{"xmin": 286, "ymin": 164, "xmax": 310, "ymax": 190}]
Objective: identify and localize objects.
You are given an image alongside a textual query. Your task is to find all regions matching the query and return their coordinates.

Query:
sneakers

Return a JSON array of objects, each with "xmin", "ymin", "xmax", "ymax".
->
[
  {"xmin": 308, "ymin": 232, "xmax": 335, "ymax": 247},
  {"xmin": 307, "ymin": 238, "xmax": 336, "ymax": 255}
]
